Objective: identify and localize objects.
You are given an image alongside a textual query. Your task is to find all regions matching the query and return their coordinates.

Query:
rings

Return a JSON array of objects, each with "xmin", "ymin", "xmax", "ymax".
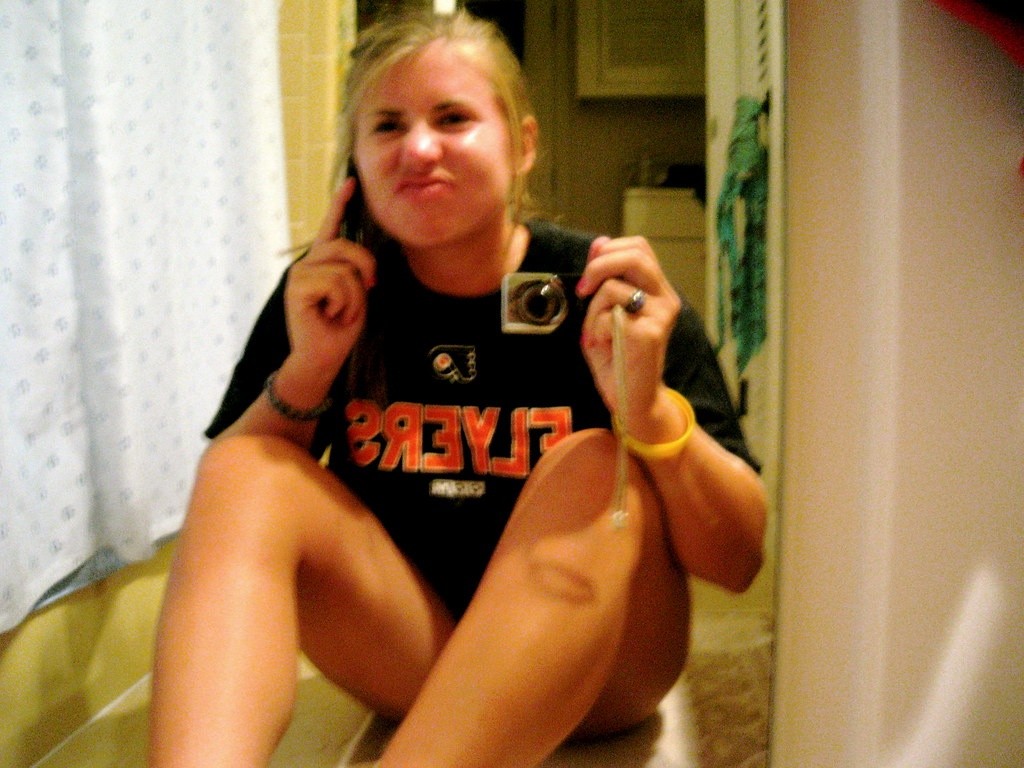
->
[{"xmin": 625, "ymin": 288, "xmax": 646, "ymax": 313}]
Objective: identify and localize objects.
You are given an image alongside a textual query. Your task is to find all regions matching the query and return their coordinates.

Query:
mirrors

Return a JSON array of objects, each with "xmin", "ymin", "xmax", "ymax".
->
[{"xmin": 0, "ymin": 0, "xmax": 790, "ymax": 768}]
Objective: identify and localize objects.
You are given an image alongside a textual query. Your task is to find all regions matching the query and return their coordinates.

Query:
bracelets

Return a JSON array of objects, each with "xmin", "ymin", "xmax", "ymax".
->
[
  {"xmin": 612, "ymin": 387, "xmax": 694, "ymax": 460},
  {"xmin": 265, "ymin": 372, "xmax": 327, "ymax": 423}
]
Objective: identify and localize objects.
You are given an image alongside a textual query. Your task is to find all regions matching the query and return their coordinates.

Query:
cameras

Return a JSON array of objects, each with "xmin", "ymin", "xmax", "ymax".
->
[{"xmin": 501, "ymin": 271, "xmax": 596, "ymax": 341}]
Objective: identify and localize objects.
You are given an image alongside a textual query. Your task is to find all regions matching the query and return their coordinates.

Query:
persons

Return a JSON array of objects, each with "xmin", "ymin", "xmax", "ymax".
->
[{"xmin": 148, "ymin": 13, "xmax": 770, "ymax": 768}]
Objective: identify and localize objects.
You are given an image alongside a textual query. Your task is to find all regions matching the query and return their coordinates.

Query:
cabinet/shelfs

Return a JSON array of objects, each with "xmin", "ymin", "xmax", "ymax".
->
[
  {"xmin": 619, "ymin": 186, "xmax": 705, "ymax": 322},
  {"xmin": 574, "ymin": 0, "xmax": 706, "ymax": 102}
]
